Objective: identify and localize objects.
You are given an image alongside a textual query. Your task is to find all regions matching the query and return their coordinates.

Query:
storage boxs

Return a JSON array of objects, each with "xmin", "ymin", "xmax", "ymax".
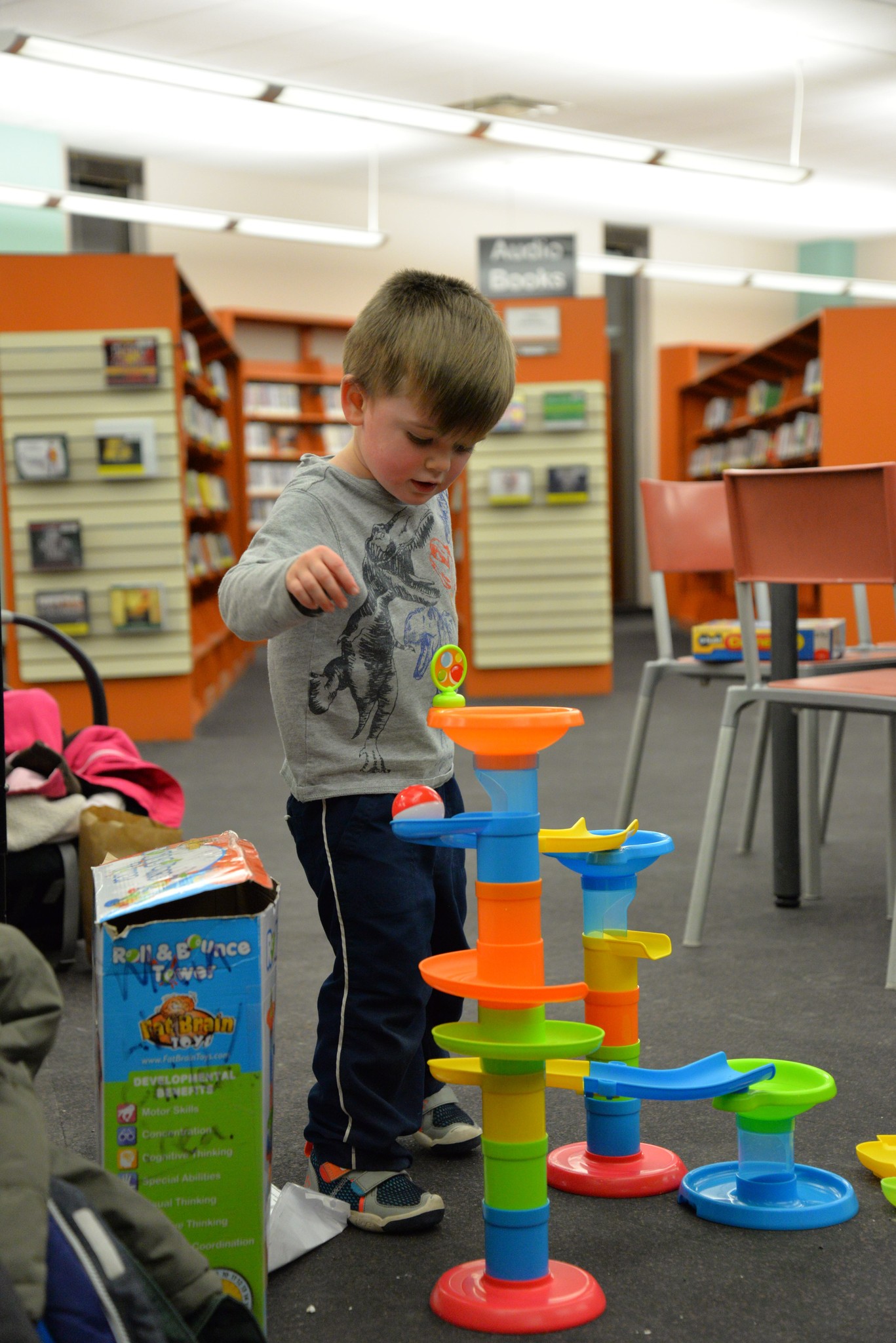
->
[
  {"xmin": 94, "ymin": 824, "xmax": 278, "ymax": 1341},
  {"xmin": 689, "ymin": 618, "xmax": 845, "ymax": 662}
]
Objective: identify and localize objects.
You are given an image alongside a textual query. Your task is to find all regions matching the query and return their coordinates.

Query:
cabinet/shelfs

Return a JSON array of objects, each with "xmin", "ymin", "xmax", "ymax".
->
[
  {"xmin": 674, "ymin": 299, "xmax": 892, "ymax": 646},
  {"xmin": 3, "ymin": 252, "xmax": 266, "ymax": 742},
  {"xmin": 207, "ymin": 308, "xmax": 363, "ymax": 550}
]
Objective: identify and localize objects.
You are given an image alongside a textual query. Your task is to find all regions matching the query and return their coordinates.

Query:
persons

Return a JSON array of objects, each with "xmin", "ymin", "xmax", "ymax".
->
[{"xmin": 219, "ymin": 265, "xmax": 527, "ymax": 1233}]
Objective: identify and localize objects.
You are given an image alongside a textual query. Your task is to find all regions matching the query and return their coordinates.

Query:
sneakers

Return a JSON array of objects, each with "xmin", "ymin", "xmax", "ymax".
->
[
  {"xmin": 409, "ymin": 1084, "xmax": 483, "ymax": 1151},
  {"xmin": 302, "ymin": 1138, "xmax": 445, "ymax": 1234}
]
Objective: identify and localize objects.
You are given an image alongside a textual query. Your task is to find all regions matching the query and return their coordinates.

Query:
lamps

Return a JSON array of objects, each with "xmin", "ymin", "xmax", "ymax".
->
[
  {"xmin": 2, "ymin": 2, "xmax": 817, "ymax": 187},
  {"xmin": 577, "ymin": 248, "xmax": 894, "ymax": 307},
  {"xmin": 0, "ymin": 127, "xmax": 388, "ymax": 252}
]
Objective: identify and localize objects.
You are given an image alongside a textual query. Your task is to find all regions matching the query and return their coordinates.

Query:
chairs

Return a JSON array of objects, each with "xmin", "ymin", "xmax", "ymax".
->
[
  {"xmin": 607, "ymin": 478, "xmax": 896, "ymax": 856},
  {"xmin": 680, "ymin": 459, "xmax": 896, "ymax": 988}
]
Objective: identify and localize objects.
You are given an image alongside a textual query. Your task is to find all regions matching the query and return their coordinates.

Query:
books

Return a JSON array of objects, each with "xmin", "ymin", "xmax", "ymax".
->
[{"xmin": 0, "ymin": 307, "xmax": 849, "ymax": 665}]
